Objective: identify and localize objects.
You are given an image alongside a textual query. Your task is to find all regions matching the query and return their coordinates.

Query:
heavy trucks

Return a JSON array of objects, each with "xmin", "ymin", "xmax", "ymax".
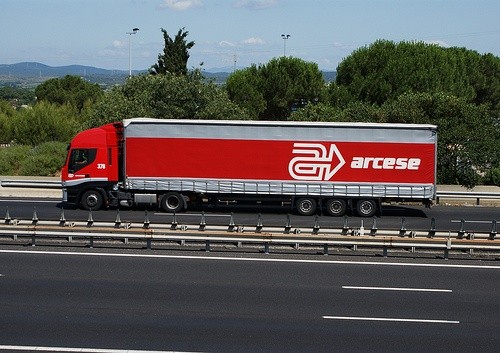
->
[{"xmin": 61, "ymin": 116, "xmax": 438, "ymax": 219}]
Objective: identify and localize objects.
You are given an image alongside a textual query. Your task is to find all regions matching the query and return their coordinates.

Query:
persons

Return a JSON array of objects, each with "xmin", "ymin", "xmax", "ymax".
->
[{"xmin": 75, "ymin": 152, "xmax": 88, "ymax": 165}]
